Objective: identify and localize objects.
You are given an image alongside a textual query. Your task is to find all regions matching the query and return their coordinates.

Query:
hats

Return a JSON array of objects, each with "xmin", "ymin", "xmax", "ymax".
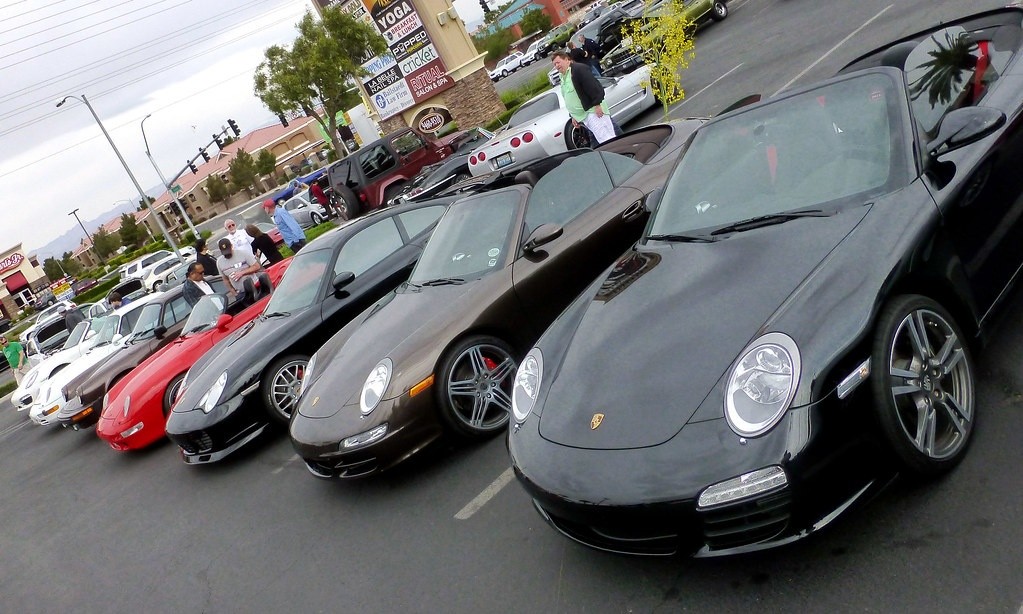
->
[
  {"xmin": 297, "ymin": 182, "xmax": 301, "ymax": 187},
  {"xmin": 219, "ymin": 238, "xmax": 232, "ymax": 255},
  {"xmin": 56, "ymin": 306, "xmax": 66, "ymax": 314},
  {"xmin": 312, "ymin": 177, "xmax": 319, "ymax": 181},
  {"xmin": 259, "ymin": 199, "xmax": 275, "ymax": 208},
  {"xmin": 566, "ymin": 41, "xmax": 573, "ymax": 50}
]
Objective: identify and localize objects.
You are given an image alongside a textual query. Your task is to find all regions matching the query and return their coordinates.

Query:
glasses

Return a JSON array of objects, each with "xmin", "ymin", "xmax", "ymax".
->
[
  {"xmin": 2, "ymin": 340, "xmax": 7, "ymax": 345},
  {"xmin": 228, "ymin": 224, "xmax": 235, "ymax": 228},
  {"xmin": 195, "ymin": 271, "xmax": 205, "ymax": 274}
]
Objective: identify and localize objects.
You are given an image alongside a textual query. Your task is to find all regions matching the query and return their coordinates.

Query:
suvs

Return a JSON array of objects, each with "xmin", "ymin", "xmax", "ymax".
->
[
  {"xmin": 118, "ymin": 246, "xmax": 196, "ymax": 280},
  {"xmin": 18, "ymin": 297, "xmax": 79, "ymax": 345},
  {"xmin": 324, "ymin": 127, "xmax": 457, "ymax": 220}
]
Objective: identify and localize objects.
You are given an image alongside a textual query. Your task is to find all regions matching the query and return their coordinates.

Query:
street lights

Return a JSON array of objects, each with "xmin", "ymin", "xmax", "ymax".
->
[
  {"xmin": 56, "ymin": 93, "xmax": 184, "ymax": 265},
  {"xmin": 68, "ymin": 209, "xmax": 106, "ymax": 262},
  {"xmin": 144, "ymin": 119, "xmax": 203, "ymax": 241},
  {"xmin": 114, "ymin": 200, "xmax": 157, "ymax": 243}
]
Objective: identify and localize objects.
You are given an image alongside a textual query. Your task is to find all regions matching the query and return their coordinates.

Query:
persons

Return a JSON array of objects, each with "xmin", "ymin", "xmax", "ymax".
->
[
  {"xmin": 182, "ymin": 262, "xmax": 224, "ymax": 321},
  {"xmin": 293, "ymin": 183, "xmax": 302, "ymax": 196},
  {"xmin": 245, "ymin": 224, "xmax": 284, "ymax": 269},
  {"xmin": 216, "ymin": 238, "xmax": 260, "ymax": 297},
  {"xmin": 551, "ymin": 51, "xmax": 616, "ymax": 144},
  {"xmin": 567, "ymin": 42, "xmax": 601, "ymax": 77},
  {"xmin": 312, "ymin": 179, "xmax": 337, "ymax": 223},
  {"xmin": 0, "ymin": 336, "xmax": 32, "ymax": 386},
  {"xmin": 262, "ymin": 199, "xmax": 306, "ymax": 254},
  {"xmin": 57, "ymin": 306, "xmax": 82, "ymax": 334},
  {"xmin": 195, "ymin": 239, "xmax": 219, "ymax": 276},
  {"xmin": 223, "ymin": 219, "xmax": 265, "ymax": 270},
  {"xmin": 578, "ymin": 35, "xmax": 605, "ymax": 75},
  {"xmin": 109, "ymin": 292, "xmax": 131, "ymax": 310}
]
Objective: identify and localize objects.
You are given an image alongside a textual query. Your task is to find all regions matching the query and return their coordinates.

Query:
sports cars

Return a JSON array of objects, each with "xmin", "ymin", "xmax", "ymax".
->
[
  {"xmin": 289, "ymin": 114, "xmax": 709, "ymax": 484},
  {"xmin": 10, "ymin": 275, "xmax": 239, "ymax": 431},
  {"xmin": 469, "ymin": 64, "xmax": 679, "ymax": 182},
  {"xmin": 165, "ymin": 192, "xmax": 488, "ymax": 466},
  {"xmin": 97, "ymin": 255, "xmax": 324, "ymax": 453},
  {"xmin": 504, "ymin": 0, "xmax": 1022, "ymax": 561}
]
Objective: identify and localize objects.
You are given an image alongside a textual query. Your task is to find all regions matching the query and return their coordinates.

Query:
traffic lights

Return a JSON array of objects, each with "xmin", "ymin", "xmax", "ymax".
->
[
  {"xmin": 213, "ymin": 134, "xmax": 224, "ymax": 150},
  {"xmin": 228, "ymin": 120, "xmax": 241, "ymax": 136},
  {"xmin": 187, "ymin": 160, "xmax": 198, "ymax": 174},
  {"xmin": 199, "ymin": 148, "xmax": 210, "ymax": 162}
]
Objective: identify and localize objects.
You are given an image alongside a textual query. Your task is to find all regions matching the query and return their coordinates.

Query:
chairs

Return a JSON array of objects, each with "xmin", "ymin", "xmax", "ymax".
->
[
  {"xmin": 256, "ymin": 274, "xmax": 274, "ymax": 298},
  {"xmin": 877, "ymin": 43, "xmax": 918, "ymax": 71},
  {"xmin": 515, "ymin": 171, "xmax": 538, "ymax": 186},
  {"xmin": 242, "ymin": 277, "xmax": 256, "ymax": 307}
]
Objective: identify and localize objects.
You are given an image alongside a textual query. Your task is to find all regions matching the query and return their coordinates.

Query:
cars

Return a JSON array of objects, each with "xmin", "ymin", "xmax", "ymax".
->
[
  {"xmin": 31, "ymin": 292, "xmax": 56, "ymax": 311},
  {"xmin": 489, "ymin": 0, "xmax": 729, "ymax": 84},
  {"xmin": 145, "ymin": 255, "xmax": 197, "ymax": 292},
  {"xmin": 271, "ymin": 167, "xmax": 328, "ymax": 206},
  {"xmin": 384, "ymin": 126, "xmax": 498, "ymax": 204},
  {"xmin": 89, "ymin": 278, "xmax": 151, "ymax": 317},
  {"xmin": 28, "ymin": 303, "xmax": 100, "ymax": 367},
  {"xmin": 283, "ymin": 189, "xmax": 336, "ymax": 232}
]
